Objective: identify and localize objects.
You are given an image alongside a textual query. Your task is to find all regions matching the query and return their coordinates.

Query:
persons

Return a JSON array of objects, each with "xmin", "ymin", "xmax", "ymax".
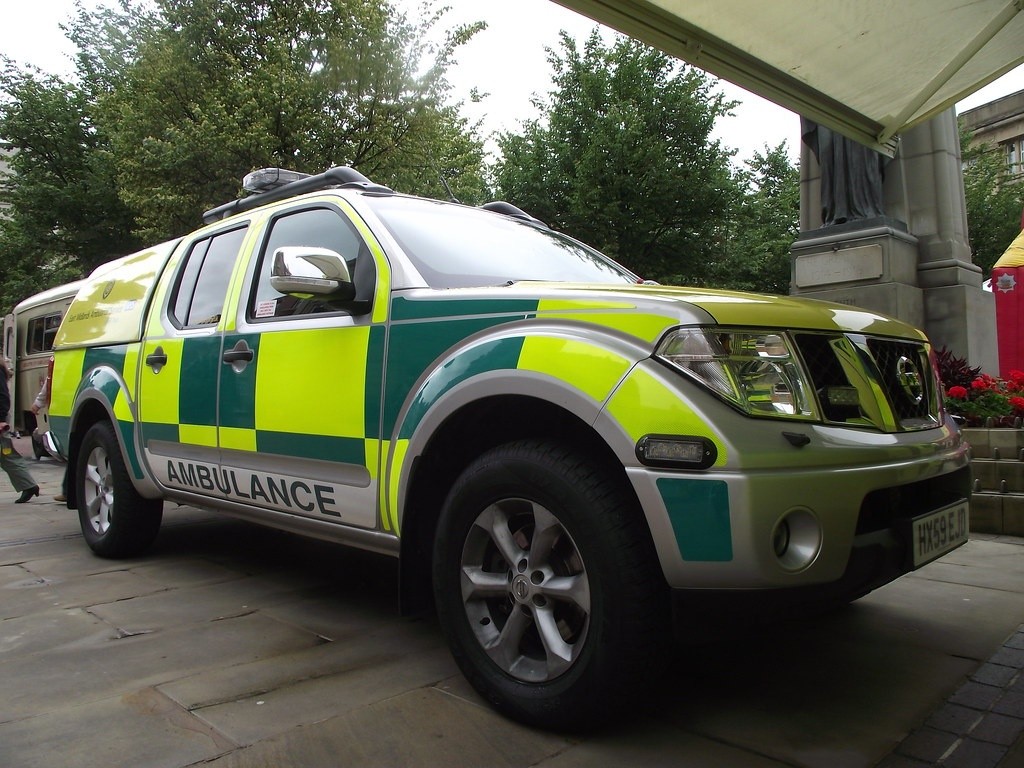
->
[
  {"xmin": 0, "ymin": 355, "xmax": 39, "ymax": 504},
  {"xmin": 31, "ymin": 370, "xmax": 68, "ymax": 503}
]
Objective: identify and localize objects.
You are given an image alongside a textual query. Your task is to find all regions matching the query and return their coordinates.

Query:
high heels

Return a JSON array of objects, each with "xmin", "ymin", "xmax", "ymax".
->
[{"xmin": 15, "ymin": 485, "xmax": 40, "ymax": 503}]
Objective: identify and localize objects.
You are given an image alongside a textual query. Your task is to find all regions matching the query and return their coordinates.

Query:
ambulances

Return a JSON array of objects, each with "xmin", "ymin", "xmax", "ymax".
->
[{"xmin": 47, "ymin": 157, "xmax": 982, "ymax": 743}]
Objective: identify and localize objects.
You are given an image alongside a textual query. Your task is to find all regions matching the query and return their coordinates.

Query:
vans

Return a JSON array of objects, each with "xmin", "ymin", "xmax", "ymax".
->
[{"xmin": 1, "ymin": 278, "xmax": 94, "ymax": 462}]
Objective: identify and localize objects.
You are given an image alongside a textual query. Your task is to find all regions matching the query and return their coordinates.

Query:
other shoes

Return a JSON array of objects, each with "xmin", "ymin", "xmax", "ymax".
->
[{"xmin": 53, "ymin": 495, "xmax": 66, "ymax": 501}]
